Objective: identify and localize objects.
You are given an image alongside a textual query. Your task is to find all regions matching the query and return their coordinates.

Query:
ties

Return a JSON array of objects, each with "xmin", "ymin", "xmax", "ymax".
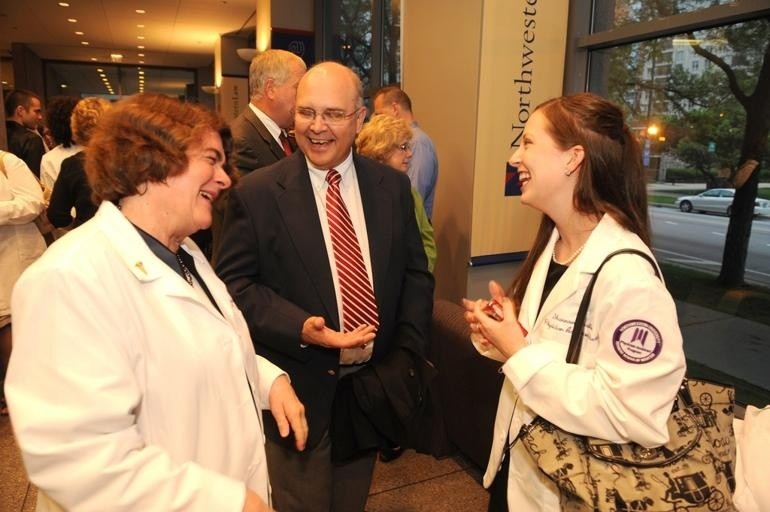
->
[{"xmin": 326, "ymin": 169, "xmax": 380, "ymax": 349}]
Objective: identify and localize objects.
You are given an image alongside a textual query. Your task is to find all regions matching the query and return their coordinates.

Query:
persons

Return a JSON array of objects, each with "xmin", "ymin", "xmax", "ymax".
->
[
  {"xmin": 459, "ymin": 92, "xmax": 690, "ymax": 511},
  {"xmin": 358, "ymin": 90, "xmax": 437, "ymax": 461},
  {"xmin": 180, "ymin": 46, "xmax": 308, "ymax": 261},
  {"xmin": 207, "ymin": 60, "xmax": 437, "ymax": 512},
  {"xmin": 3, "ymin": 91, "xmax": 311, "ymax": 512},
  {"xmin": 1, "ymin": 90, "xmax": 111, "ymax": 409}
]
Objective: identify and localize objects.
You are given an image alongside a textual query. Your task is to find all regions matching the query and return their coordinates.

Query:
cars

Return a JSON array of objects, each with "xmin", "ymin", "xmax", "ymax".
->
[{"xmin": 675, "ymin": 188, "xmax": 769, "ymax": 219}]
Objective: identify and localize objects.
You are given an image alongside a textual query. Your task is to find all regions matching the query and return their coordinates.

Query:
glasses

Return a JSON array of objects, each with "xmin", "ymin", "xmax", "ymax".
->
[
  {"xmin": 289, "ymin": 107, "xmax": 361, "ymax": 124},
  {"xmin": 396, "ymin": 142, "xmax": 415, "ymax": 152}
]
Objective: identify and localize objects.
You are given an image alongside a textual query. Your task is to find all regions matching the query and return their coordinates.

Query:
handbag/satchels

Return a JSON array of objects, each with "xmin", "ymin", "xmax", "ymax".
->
[{"xmin": 518, "ymin": 379, "xmax": 736, "ymax": 510}]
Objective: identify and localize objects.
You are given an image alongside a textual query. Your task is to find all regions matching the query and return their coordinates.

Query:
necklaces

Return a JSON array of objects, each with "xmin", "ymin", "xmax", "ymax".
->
[
  {"xmin": 176, "ymin": 252, "xmax": 194, "ymax": 287},
  {"xmin": 550, "ymin": 217, "xmax": 611, "ymax": 265}
]
